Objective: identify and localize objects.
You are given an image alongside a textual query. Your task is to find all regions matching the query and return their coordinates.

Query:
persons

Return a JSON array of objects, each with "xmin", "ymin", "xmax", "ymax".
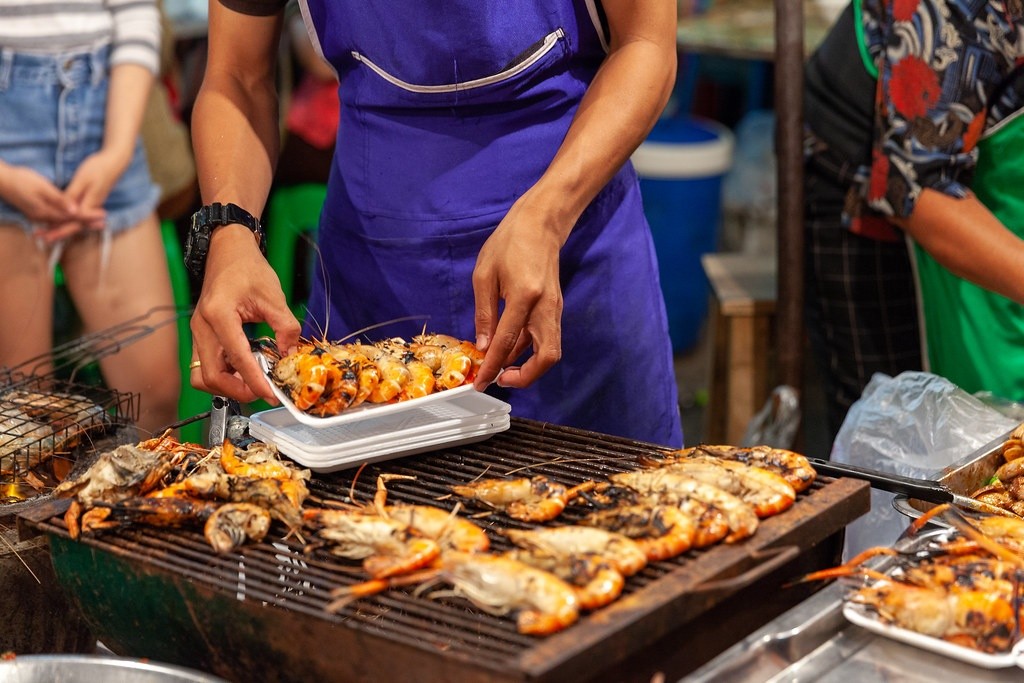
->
[
  {"xmin": 184, "ymin": 0, "xmax": 685, "ymax": 450},
  {"xmin": 772, "ymin": 0, "xmax": 1024, "ymax": 451},
  {"xmin": 0, "ymin": 0, "xmax": 181, "ymax": 442},
  {"xmin": 154, "ymin": 8, "xmax": 339, "ymax": 221}
]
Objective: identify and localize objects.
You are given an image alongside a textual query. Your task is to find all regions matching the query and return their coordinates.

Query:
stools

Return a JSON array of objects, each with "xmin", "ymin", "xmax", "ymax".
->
[
  {"xmin": 53, "ymin": 184, "xmax": 327, "ymax": 445},
  {"xmin": 700, "ymin": 252, "xmax": 779, "ymax": 446}
]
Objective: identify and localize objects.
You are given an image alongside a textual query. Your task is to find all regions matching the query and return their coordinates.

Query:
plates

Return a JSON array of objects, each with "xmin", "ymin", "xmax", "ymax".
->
[
  {"xmin": 248, "ymin": 353, "xmax": 511, "ymax": 473},
  {"xmin": 843, "ymin": 532, "xmax": 1024, "ymax": 669}
]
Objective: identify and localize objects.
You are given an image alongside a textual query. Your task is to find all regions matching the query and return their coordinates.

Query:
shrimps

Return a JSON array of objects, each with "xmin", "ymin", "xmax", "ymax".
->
[
  {"xmin": 64, "ymin": 428, "xmax": 816, "ymax": 633},
  {"xmin": 273, "ymin": 334, "xmax": 487, "ymax": 415},
  {"xmin": 793, "ymin": 503, "xmax": 1024, "ymax": 659}
]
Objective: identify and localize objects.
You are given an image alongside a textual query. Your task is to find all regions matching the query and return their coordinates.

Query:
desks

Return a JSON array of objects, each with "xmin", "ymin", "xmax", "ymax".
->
[{"xmin": 677, "ymin": 1, "xmax": 851, "ymax": 63}]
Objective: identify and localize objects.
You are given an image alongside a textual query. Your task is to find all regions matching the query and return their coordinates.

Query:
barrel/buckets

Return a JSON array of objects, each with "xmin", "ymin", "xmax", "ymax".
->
[{"xmin": 627, "ymin": 119, "xmax": 734, "ymax": 359}]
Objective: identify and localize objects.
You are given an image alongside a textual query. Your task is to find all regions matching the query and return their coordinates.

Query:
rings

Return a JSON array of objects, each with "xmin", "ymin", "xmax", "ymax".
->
[{"xmin": 189, "ymin": 361, "xmax": 201, "ymax": 369}]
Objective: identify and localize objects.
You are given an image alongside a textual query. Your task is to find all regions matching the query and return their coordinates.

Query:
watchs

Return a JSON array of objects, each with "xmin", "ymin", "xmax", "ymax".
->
[{"xmin": 184, "ymin": 202, "xmax": 263, "ymax": 281}]
